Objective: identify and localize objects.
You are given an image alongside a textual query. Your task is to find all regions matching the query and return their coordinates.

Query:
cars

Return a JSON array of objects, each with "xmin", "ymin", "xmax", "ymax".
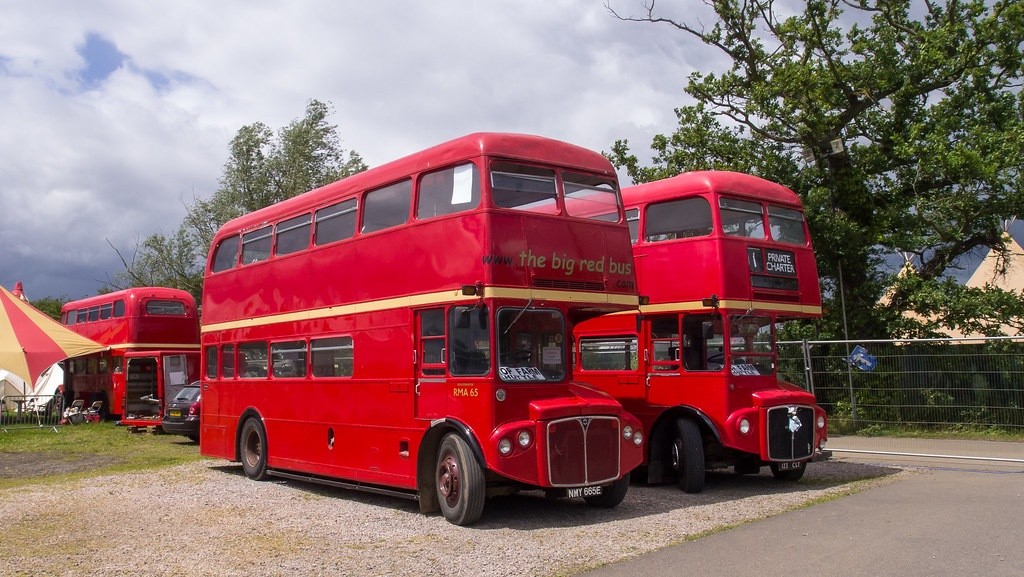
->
[{"xmin": 161, "ymin": 380, "xmax": 200, "ymax": 443}]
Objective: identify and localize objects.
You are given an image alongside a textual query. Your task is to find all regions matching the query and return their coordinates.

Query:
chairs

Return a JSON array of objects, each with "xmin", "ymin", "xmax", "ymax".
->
[
  {"xmin": 63, "ymin": 400, "xmax": 85, "ymax": 426},
  {"xmin": 298, "ymin": 346, "xmax": 335, "ymax": 376},
  {"xmin": 667, "ymin": 346, "xmax": 704, "ymax": 370},
  {"xmin": 81, "ymin": 401, "xmax": 103, "ymax": 423},
  {"xmin": 452, "ymin": 349, "xmax": 488, "ymax": 375}
]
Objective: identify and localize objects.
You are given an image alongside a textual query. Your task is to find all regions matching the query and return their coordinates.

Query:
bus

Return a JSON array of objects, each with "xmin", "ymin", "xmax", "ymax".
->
[
  {"xmin": 60, "ymin": 286, "xmax": 201, "ymax": 421},
  {"xmin": 199, "ymin": 131, "xmax": 650, "ymax": 526},
  {"xmin": 508, "ymin": 171, "xmax": 833, "ymax": 494}
]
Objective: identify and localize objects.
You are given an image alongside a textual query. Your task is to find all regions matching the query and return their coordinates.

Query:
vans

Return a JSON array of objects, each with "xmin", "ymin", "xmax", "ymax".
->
[{"xmin": 120, "ymin": 351, "xmax": 201, "ymax": 434}]
